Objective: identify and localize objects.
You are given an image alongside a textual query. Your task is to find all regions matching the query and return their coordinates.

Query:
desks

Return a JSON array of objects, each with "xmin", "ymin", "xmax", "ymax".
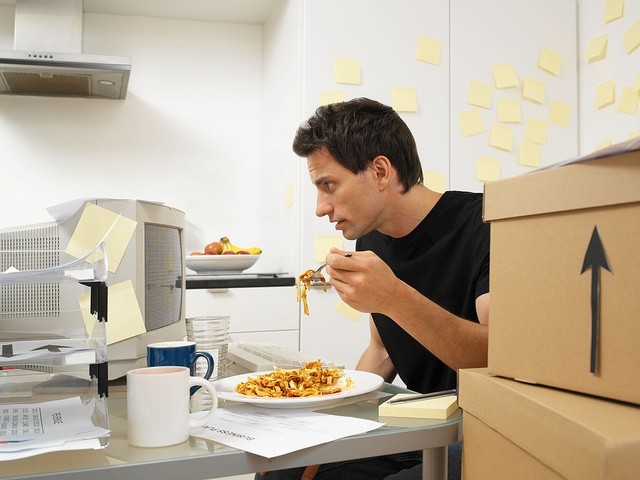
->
[{"xmin": 2, "ymin": 346, "xmax": 462, "ymax": 478}]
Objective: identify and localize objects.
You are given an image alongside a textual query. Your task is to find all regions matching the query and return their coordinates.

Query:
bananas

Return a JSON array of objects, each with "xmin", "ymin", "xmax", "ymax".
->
[{"xmin": 220, "ymin": 237, "xmax": 263, "ymax": 257}]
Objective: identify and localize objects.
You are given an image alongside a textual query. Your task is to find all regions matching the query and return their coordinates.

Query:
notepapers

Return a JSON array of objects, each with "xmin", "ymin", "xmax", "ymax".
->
[{"xmin": 378, "ymin": 393, "xmax": 459, "ymax": 420}]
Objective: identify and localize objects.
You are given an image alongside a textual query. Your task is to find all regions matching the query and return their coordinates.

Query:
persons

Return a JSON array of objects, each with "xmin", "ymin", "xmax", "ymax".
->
[{"xmin": 252, "ymin": 96, "xmax": 490, "ymax": 479}]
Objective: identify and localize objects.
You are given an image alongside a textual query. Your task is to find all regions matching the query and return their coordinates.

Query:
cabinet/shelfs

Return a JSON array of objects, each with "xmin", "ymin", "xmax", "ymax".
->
[
  {"xmin": 577, "ymin": 0, "xmax": 640, "ymax": 160},
  {"xmin": 305, "ymin": 1, "xmax": 578, "ymax": 286},
  {"xmin": 185, "ymin": 287, "xmax": 301, "ymax": 354}
]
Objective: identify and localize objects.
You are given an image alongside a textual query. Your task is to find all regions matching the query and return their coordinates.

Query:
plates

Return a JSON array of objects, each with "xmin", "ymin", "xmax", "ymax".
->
[
  {"xmin": 183, "ymin": 253, "xmax": 262, "ymax": 275},
  {"xmin": 208, "ymin": 369, "xmax": 385, "ymax": 407}
]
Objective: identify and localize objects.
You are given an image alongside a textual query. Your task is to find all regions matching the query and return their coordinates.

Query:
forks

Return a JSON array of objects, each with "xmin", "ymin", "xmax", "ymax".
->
[{"xmin": 303, "ymin": 254, "xmax": 354, "ymax": 282}]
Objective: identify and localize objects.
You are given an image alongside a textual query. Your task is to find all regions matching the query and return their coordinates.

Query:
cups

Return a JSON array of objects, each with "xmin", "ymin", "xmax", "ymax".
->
[
  {"xmin": 146, "ymin": 341, "xmax": 213, "ymax": 399},
  {"xmin": 126, "ymin": 366, "xmax": 218, "ymax": 449},
  {"xmin": 184, "ymin": 316, "xmax": 232, "ymax": 381}
]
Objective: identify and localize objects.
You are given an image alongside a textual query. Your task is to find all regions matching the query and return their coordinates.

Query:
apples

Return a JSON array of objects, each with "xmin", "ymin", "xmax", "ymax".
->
[{"xmin": 205, "ymin": 242, "xmax": 222, "ymax": 254}]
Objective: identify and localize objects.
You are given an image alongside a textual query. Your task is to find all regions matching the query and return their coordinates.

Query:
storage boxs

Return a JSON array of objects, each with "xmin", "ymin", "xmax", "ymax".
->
[
  {"xmin": 482, "ymin": 152, "xmax": 639, "ymax": 409},
  {"xmin": 456, "ymin": 367, "xmax": 640, "ymax": 478}
]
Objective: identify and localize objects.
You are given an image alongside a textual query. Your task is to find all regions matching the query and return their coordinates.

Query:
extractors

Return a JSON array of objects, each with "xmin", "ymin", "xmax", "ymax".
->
[{"xmin": 0, "ymin": 1, "xmax": 133, "ymax": 102}]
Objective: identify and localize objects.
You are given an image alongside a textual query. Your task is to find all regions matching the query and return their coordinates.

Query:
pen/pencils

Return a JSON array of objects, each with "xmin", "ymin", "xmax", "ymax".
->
[{"xmin": 386, "ymin": 389, "xmax": 457, "ymax": 403}]
